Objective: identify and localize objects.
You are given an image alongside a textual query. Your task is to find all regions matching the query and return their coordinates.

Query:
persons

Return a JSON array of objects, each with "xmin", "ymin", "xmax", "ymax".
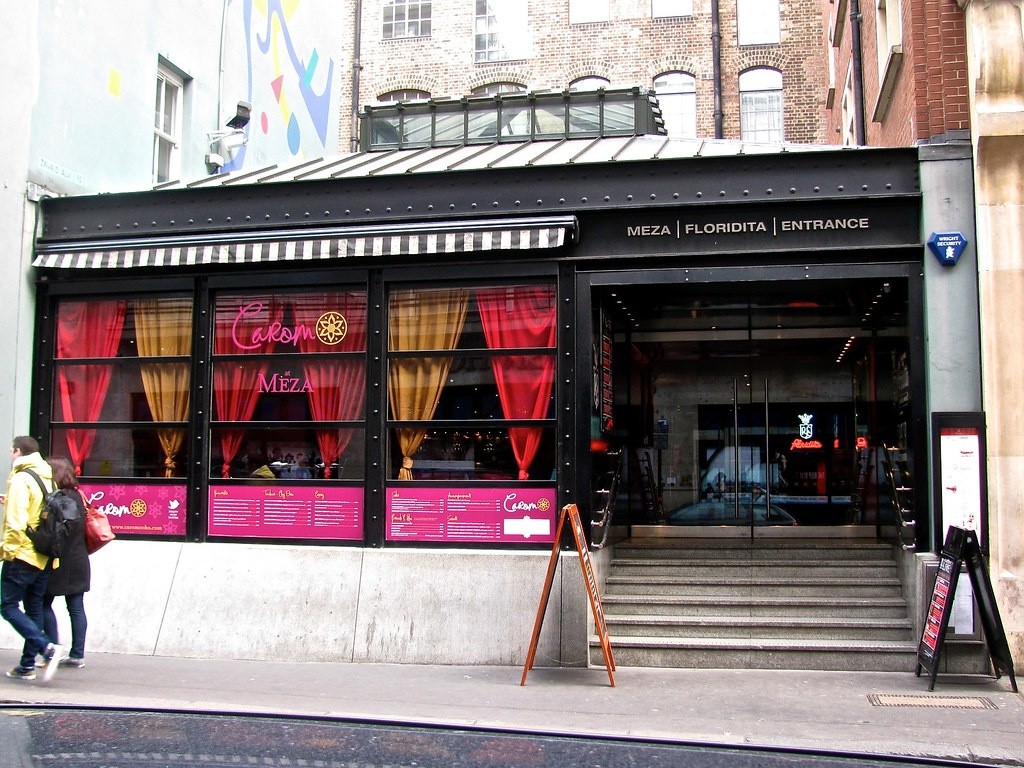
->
[
  {"xmin": 34, "ymin": 455, "xmax": 90, "ymax": 669},
  {"xmin": 245, "ymin": 454, "xmax": 274, "ymax": 479},
  {"xmin": 0, "ymin": 436, "xmax": 63, "ymax": 681}
]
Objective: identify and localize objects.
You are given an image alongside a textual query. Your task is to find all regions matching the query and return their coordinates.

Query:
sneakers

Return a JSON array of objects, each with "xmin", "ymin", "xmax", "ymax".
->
[
  {"xmin": 6, "ymin": 664, "xmax": 36, "ymax": 680},
  {"xmin": 42, "ymin": 644, "xmax": 63, "ymax": 681}
]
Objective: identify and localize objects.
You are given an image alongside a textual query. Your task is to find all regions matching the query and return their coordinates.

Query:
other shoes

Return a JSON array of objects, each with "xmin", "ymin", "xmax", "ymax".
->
[
  {"xmin": 58, "ymin": 656, "xmax": 86, "ymax": 668},
  {"xmin": 34, "ymin": 651, "xmax": 66, "ymax": 666}
]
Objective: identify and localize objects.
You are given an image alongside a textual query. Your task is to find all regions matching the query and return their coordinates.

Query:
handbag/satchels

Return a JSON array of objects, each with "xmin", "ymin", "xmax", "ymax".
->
[{"xmin": 72, "ymin": 486, "xmax": 115, "ymax": 556}]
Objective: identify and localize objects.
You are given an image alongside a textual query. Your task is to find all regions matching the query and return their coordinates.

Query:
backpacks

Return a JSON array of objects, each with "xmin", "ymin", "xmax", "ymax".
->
[{"xmin": 15, "ymin": 468, "xmax": 81, "ymax": 558}]
